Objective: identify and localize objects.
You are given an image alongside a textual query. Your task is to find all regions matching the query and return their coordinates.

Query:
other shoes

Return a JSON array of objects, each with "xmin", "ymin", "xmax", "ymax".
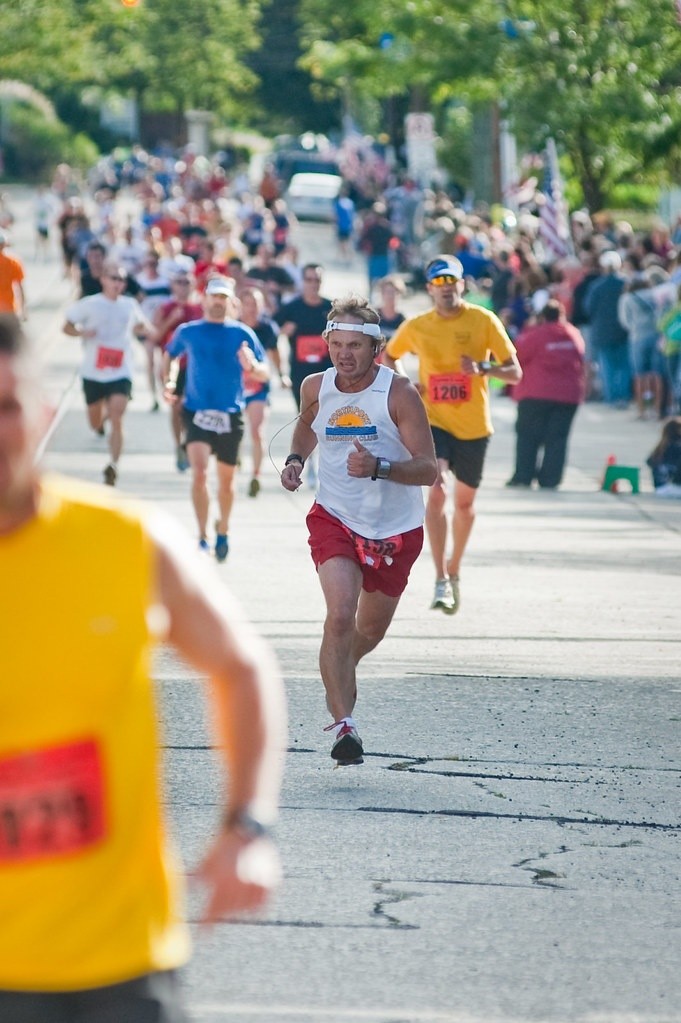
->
[
  {"xmin": 178, "ymin": 457, "xmax": 189, "ymax": 469},
  {"xmin": 249, "ymin": 478, "xmax": 260, "ymax": 498},
  {"xmin": 105, "ymin": 465, "xmax": 116, "ymax": 486},
  {"xmin": 216, "ymin": 532, "xmax": 228, "ymax": 561},
  {"xmin": 199, "ymin": 539, "xmax": 209, "ymax": 551}
]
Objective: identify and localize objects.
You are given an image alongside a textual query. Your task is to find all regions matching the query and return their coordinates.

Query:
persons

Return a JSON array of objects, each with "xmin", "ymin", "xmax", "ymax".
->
[
  {"xmin": 647, "ymin": 419, "xmax": 681, "ymax": 495},
  {"xmin": 383, "ymin": 255, "xmax": 522, "ymax": 614},
  {"xmin": 29, "ymin": 139, "xmax": 409, "ymax": 498},
  {"xmin": 159, "ymin": 277, "xmax": 272, "ymax": 559},
  {"xmin": 0, "ymin": 312, "xmax": 287, "ymax": 1023},
  {"xmin": 507, "ymin": 300, "xmax": 586, "ymax": 488},
  {"xmin": 0, "ymin": 232, "xmax": 27, "ymax": 321},
  {"xmin": 280, "ymin": 295, "xmax": 439, "ymax": 767},
  {"xmin": 354, "ymin": 176, "xmax": 681, "ymax": 422}
]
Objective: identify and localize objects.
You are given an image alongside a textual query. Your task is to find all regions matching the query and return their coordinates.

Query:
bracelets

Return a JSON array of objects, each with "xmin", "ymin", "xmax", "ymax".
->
[
  {"xmin": 250, "ymin": 362, "xmax": 255, "ymax": 374},
  {"xmin": 222, "ymin": 812, "xmax": 276, "ymax": 839},
  {"xmin": 285, "ymin": 454, "xmax": 304, "ymax": 469}
]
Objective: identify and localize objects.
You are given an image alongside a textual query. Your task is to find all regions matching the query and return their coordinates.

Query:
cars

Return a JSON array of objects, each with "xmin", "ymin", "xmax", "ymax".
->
[{"xmin": 284, "ymin": 172, "xmax": 353, "ymax": 225}]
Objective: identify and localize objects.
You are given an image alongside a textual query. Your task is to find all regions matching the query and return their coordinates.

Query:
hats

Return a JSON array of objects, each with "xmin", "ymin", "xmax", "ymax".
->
[
  {"xmin": 206, "ymin": 279, "xmax": 234, "ymax": 298},
  {"xmin": 424, "ymin": 260, "xmax": 462, "ymax": 281}
]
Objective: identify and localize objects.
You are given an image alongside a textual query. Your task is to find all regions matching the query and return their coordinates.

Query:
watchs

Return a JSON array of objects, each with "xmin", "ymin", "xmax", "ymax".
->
[
  {"xmin": 376, "ymin": 456, "xmax": 391, "ymax": 480},
  {"xmin": 480, "ymin": 359, "xmax": 490, "ymax": 375}
]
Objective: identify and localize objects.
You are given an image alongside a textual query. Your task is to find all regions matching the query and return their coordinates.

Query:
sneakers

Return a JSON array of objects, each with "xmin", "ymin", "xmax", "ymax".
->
[
  {"xmin": 431, "ymin": 578, "xmax": 455, "ymax": 610},
  {"xmin": 442, "ymin": 570, "xmax": 461, "ymax": 615},
  {"xmin": 330, "ymin": 725, "xmax": 364, "ymax": 766}
]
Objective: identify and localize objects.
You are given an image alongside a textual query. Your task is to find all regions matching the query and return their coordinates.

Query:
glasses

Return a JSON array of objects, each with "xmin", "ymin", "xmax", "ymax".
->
[
  {"xmin": 430, "ymin": 275, "xmax": 459, "ymax": 287},
  {"xmin": 105, "ymin": 274, "xmax": 125, "ymax": 281}
]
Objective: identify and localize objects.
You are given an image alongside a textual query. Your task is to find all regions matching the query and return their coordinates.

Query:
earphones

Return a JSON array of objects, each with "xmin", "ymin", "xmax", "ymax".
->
[{"xmin": 373, "ymin": 345, "xmax": 379, "ymax": 356}]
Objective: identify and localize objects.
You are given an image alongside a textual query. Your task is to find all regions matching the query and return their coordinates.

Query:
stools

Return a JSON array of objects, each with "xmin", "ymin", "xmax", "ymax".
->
[{"xmin": 602, "ymin": 464, "xmax": 642, "ymax": 494}]
